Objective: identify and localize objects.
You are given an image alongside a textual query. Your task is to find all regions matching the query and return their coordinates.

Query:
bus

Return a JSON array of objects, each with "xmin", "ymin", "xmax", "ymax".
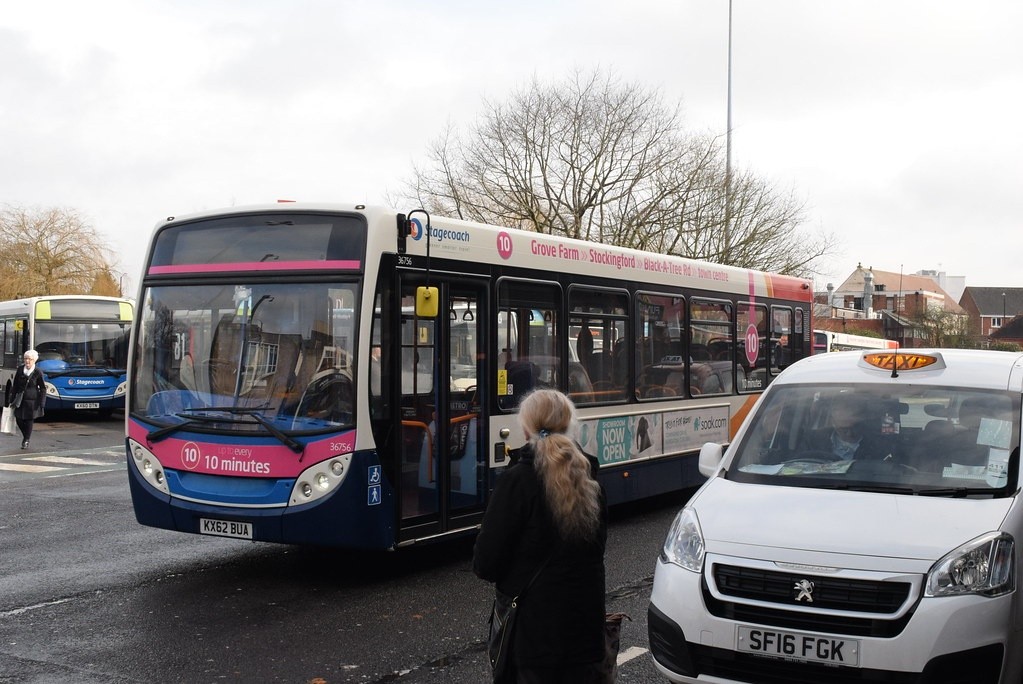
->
[
  {"xmin": 451, "ymin": 305, "xmax": 549, "ymax": 392},
  {"xmin": 782, "ymin": 327, "xmax": 900, "ymax": 355},
  {"xmin": 567, "ymin": 326, "xmax": 619, "ymax": 364},
  {"xmin": 144, "ymin": 305, "xmax": 434, "ymax": 393},
  {"xmin": 0, "ymin": 295, "xmax": 134, "ymax": 412},
  {"xmin": 124, "ymin": 200, "xmax": 818, "ymax": 555}
]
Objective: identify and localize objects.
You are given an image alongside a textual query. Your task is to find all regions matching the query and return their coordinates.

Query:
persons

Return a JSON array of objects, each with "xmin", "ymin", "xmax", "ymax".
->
[
  {"xmin": 472, "ymin": 390, "xmax": 607, "ymax": 684},
  {"xmin": 933, "ymin": 403, "xmax": 1020, "ymax": 496},
  {"xmin": 372, "ymin": 346, "xmax": 380, "ymax": 396},
  {"xmin": 793, "ymin": 399, "xmax": 881, "ymax": 462},
  {"xmin": 640, "ymin": 369, "xmax": 667, "ymax": 395},
  {"xmin": 9, "ymin": 350, "xmax": 47, "ymax": 449},
  {"xmin": 500, "ymin": 361, "xmax": 540, "ymax": 409}
]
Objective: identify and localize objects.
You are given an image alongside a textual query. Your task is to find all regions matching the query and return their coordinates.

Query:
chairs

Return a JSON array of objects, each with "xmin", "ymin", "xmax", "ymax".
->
[
  {"xmin": 586, "ymin": 336, "xmax": 784, "ymax": 403},
  {"xmin": 932, "ymin": 395, "xmax": 1001, "ymax": 482}
]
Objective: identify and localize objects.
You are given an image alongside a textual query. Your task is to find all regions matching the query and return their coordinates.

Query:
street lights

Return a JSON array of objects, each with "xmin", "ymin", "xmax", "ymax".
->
[
  {"xmin": 1002, "ymin": 293, "xmax": 1006, "ymax": 324},
  {"xmin": 896, "ymin": 264, "xmax": 904, "ymax": 339}
]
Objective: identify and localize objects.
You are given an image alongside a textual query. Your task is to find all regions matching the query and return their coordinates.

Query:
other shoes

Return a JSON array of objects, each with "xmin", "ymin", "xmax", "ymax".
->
[{"xmin": 21, "ymin": 439, "xmax": 29, "ymax": 449}]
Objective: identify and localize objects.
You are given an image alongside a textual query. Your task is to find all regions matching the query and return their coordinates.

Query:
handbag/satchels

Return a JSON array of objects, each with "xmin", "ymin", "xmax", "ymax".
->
[
  {"xmin": 486, "ymin": 584, "xmax": 518, "ymax": 678},
  {"xmin": 605, "ymin": 610, "xmax": 634, "ymax": 682},
  {"xmin": 10, "ymin": 391, "xmax": 23, "ymax": 408},
  {"xmin": 1, "ymin": 406, "xmax": 17, "ymax": 436}
]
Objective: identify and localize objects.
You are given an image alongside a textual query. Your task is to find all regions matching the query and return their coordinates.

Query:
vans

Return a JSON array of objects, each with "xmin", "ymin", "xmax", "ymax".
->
[{"xmin": 646, "ymin": 349, "xmax": 1023, "ymax": 684}]
